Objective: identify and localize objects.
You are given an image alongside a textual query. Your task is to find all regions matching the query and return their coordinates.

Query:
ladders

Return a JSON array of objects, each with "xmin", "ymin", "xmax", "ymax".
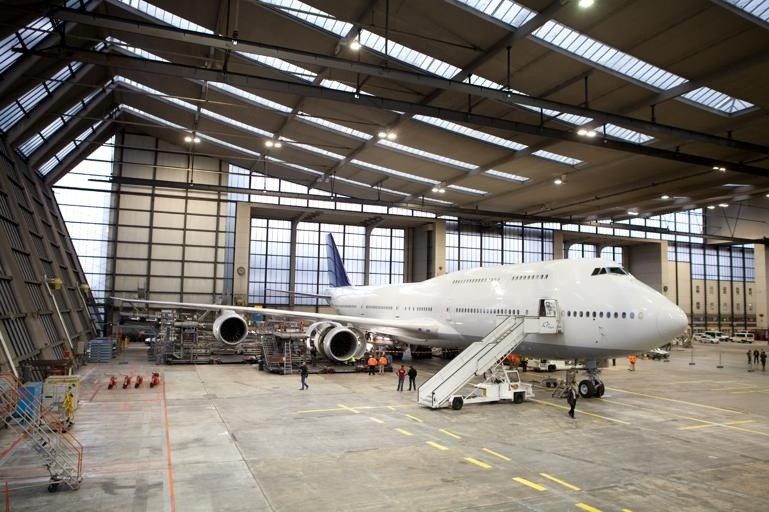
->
[{"xmin": 283, "ymin": 340, "xmax": 293, "ymax": 375}]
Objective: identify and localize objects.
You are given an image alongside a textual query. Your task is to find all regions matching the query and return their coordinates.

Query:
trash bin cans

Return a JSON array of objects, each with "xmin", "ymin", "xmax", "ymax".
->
[{"xmin": 156, "ymin": 354, "xmax": 164, "ymax": 364}]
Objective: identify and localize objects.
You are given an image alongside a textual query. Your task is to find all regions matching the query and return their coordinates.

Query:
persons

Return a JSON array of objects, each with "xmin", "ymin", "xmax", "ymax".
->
[
  {"xmin": 567, "ymin": 384, "xmax": 580, "ymax": 419},
  {"xmin": 520, "ymin": 355, "xmax": 528, "ymax": 373},
  {"xmin": 379, "ymin": 354, "xmax": 388, "ymax": 375},
  {"xmin": 299, "ymin": 362, "xmax": 309, "ymax": 390},
  {"xmin": 629, "ymin": 354, "xmax": 636, "ymax": 372},
  {"xmin": 746, "ymin": 348, "xmax": 767, "ymax": 372},
  {"xmin": 408, "ymin": 366, "xmax": 417, "ymax": 390},
  {"xmin": 395, "ymin": 364, "xmax": 407, "ymax": 391},
  {"xmin": 368, "ymin": 355, "xmax": 377, "ymax": 376}
]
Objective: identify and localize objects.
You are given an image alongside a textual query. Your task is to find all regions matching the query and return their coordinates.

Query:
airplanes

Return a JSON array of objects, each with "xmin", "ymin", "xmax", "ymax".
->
[{"xmin": 108, "ymin": 232, "xmax": 689, "ymax": 410}]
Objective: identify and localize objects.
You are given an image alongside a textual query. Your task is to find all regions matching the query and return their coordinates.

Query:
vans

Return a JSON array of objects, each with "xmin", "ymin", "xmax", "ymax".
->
[{"xmin": 693, "ymin": 331, "xmax": 755, "ymax": 343}]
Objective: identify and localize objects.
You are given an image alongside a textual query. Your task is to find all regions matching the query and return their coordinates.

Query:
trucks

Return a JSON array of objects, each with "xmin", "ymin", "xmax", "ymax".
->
[{"xmin": 448, "ymin": 362, "xmax": 536, "ymax": 410}]
